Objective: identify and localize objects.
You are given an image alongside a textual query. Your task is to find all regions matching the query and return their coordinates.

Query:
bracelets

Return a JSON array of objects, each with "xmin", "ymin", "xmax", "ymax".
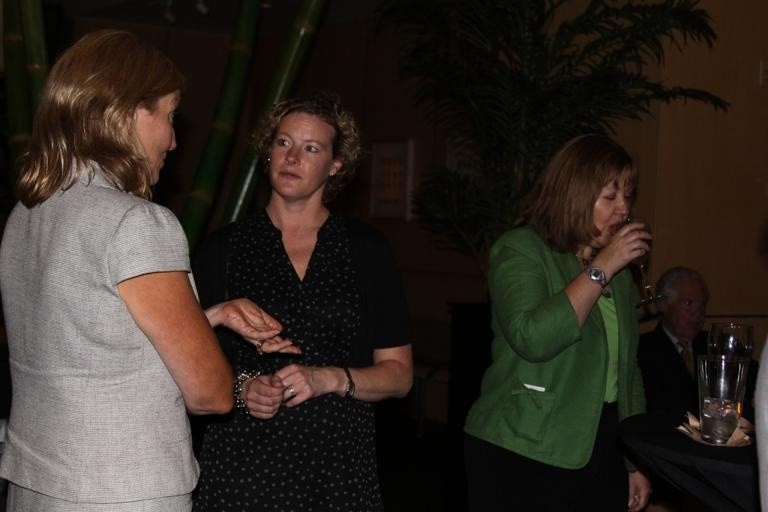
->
[{"xmin": 232, "ymin": 368, "xmax": 264, "ymax": 419}]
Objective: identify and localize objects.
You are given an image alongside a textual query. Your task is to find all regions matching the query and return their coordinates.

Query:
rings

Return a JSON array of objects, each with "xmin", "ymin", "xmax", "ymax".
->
[
  {"xmin": 255, "ymin": 339, "xmax": 264, "ymax": 355},
  {"xmin": 288, "ymin": 384, "xmax": 296, "ymax": 396}
]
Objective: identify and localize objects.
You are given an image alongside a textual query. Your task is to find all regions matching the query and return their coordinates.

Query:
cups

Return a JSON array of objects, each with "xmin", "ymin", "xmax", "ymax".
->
[
  {"xmin": 707, "ymin": 320, "xmax": 755, "ymax": 362},
  {"xmin": 698, "ymin": 353, "xmax": 749, "ymax": 446}
]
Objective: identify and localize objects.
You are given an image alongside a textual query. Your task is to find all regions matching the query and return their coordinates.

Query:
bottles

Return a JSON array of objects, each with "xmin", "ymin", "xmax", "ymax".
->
[{"xmin": 376, "ymin": 158, "xmax": 402, "ymax": 192}]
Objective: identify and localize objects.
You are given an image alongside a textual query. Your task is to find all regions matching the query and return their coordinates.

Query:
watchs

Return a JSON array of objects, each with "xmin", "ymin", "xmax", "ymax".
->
[
  {"xmin": 584, "ymin": 265, "xmax": 608, "ymax": 288},
  {"xmin": 342, "ymin": 367, "xmax": 355, "ymax": 401}
]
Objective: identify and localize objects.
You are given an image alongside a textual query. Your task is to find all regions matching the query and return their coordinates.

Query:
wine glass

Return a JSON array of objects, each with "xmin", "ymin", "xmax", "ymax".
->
[{"xmin": 614, "ymin": 218, "xmax": 666, "ymax": 309}]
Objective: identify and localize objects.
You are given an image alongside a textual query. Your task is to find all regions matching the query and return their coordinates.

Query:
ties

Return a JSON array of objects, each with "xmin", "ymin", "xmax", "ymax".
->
[{"xmin": 677, "ymin": 341, "xmax": 693, "ymax": 374}]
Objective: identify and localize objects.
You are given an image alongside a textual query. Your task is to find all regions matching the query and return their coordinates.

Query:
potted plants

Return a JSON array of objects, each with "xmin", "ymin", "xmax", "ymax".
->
[{"xmin": 372, "ymin": 0, "xmax": 732, "ymax": 435}]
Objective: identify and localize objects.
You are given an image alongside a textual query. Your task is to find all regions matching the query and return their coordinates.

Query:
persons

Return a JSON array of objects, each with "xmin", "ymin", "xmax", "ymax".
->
[
  {"xmin": 189, "ymin": 86, "xmax": 415, "ymax": 509},
  {"xmin": 462, "ymin": 131, "xmax": 654, "ymax": 511},
  {"xmin": 0, "ymin": 27, "xmax": 303, "ymax": 511},
  {"xmin": 753, "ymin": 336, "xmax": 768, "ymax": 512},
  {"xmin": 637, "ymin": 266, "xmax": 758, "ymax": 412}
]
